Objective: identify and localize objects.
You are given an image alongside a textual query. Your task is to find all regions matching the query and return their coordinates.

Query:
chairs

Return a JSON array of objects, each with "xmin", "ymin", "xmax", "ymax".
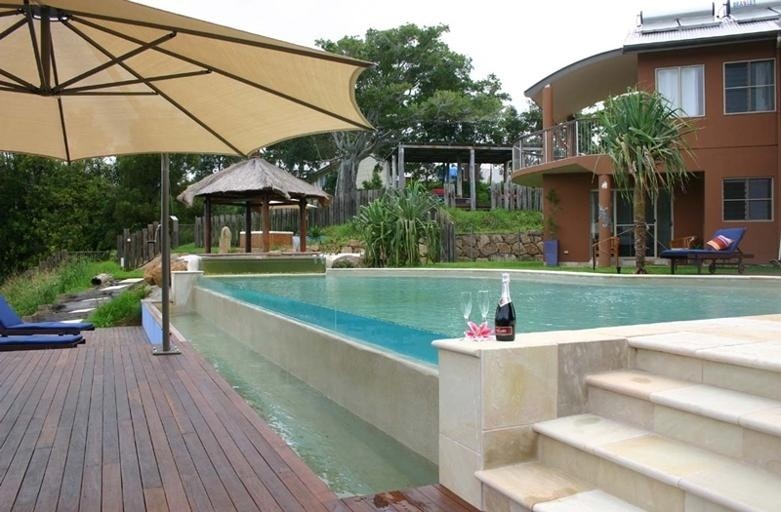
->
[
  {"xmin": 659, "ymin": 227, "xmax": 755, "ymax": 274},
  {"xmin": 668, "ymin": 235, "xmax": 698, "ymax": 263},
  {"xmin": 0, "ymin": 294, "xmax": 96, "ymax": 351}
]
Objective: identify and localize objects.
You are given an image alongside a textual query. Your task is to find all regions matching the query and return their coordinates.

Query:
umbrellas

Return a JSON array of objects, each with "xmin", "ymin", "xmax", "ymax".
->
[{"xmin": 0, "ymin": 0, "xmax": 380, "ymax": 168}]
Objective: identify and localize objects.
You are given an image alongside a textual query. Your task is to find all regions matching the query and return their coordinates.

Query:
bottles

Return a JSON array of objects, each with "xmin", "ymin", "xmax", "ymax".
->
[{"xmin": 495, "ymin": 273, "xmax": 517, "ymax": 341}]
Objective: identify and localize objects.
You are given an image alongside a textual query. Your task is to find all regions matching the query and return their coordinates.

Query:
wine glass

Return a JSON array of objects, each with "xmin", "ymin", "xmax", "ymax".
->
[
  {"xmin": 458, "ymin": 291, "xmax": 473, "ymax": 342},
  {"xmin": 477, "ymin": 291, "xmax": 490, "ymax": 321}
]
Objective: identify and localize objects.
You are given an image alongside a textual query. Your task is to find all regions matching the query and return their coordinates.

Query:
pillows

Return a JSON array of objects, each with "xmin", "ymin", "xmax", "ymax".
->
[{"xmin": 705, "ymin": 234, "xmax": 736, "ymax": 252}]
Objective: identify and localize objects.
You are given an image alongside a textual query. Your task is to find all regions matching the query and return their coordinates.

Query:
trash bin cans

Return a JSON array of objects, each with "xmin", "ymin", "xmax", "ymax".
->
[{"xmin": 543, "ymin": 240, "xmax": 559, "ymax": 266}]
